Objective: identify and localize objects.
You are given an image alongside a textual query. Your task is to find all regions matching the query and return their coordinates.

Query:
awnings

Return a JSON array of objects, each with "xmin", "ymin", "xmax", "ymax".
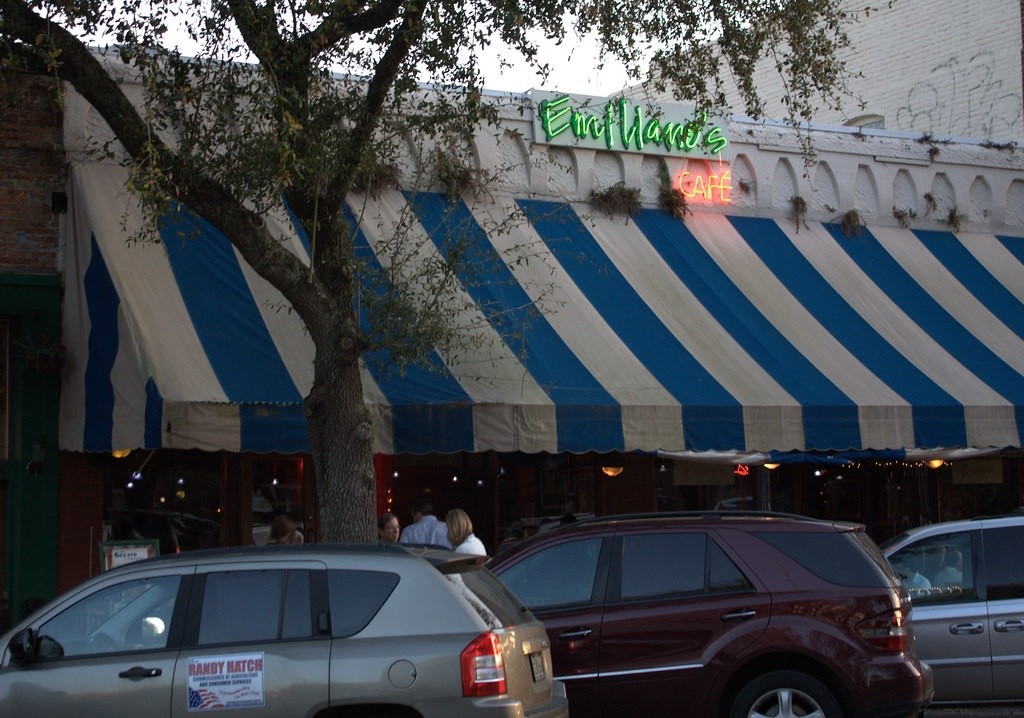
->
[{"xmin": 58, "ymin": 160, "xmax": 1024, "ymax": 454}]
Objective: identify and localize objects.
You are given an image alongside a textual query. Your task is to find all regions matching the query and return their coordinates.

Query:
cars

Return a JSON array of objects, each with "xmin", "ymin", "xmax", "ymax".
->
[
  {"xmin": 0, "ymin": 548, "xmax": 573, "ymax": 717},
  {"xmin": 819, "ymin": 517, "xmax": 1024, "ymax": 703}
]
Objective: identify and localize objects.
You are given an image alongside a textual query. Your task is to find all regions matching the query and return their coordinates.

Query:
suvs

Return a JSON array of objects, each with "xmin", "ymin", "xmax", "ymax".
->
[{"xmin": 460, "ymin": 503, "xmax": 939, "ymax": 718}]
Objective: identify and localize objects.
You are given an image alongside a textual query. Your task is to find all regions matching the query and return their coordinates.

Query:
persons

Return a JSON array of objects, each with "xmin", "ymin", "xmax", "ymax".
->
[
  {"xmin": 894, "ymin": 549, "xmax": 963, "ymax": 589},
  {"xmin": 445, "ymin": 508, "xmax": 487, "ymax": 556},
  {"xmin": 399, "ymin": 497, "xmax": 453, "ymax": 549},
  {"xmin": 377, "ymin": 513, "xmax": 399, "ymax": 544},
  {"xmin": 257, "ymin": 514, "xmax": 304, "ymax": 546},
  {"xmin": 539, "ymin": 514, "xmax": 581, "ymax": 537}
]
